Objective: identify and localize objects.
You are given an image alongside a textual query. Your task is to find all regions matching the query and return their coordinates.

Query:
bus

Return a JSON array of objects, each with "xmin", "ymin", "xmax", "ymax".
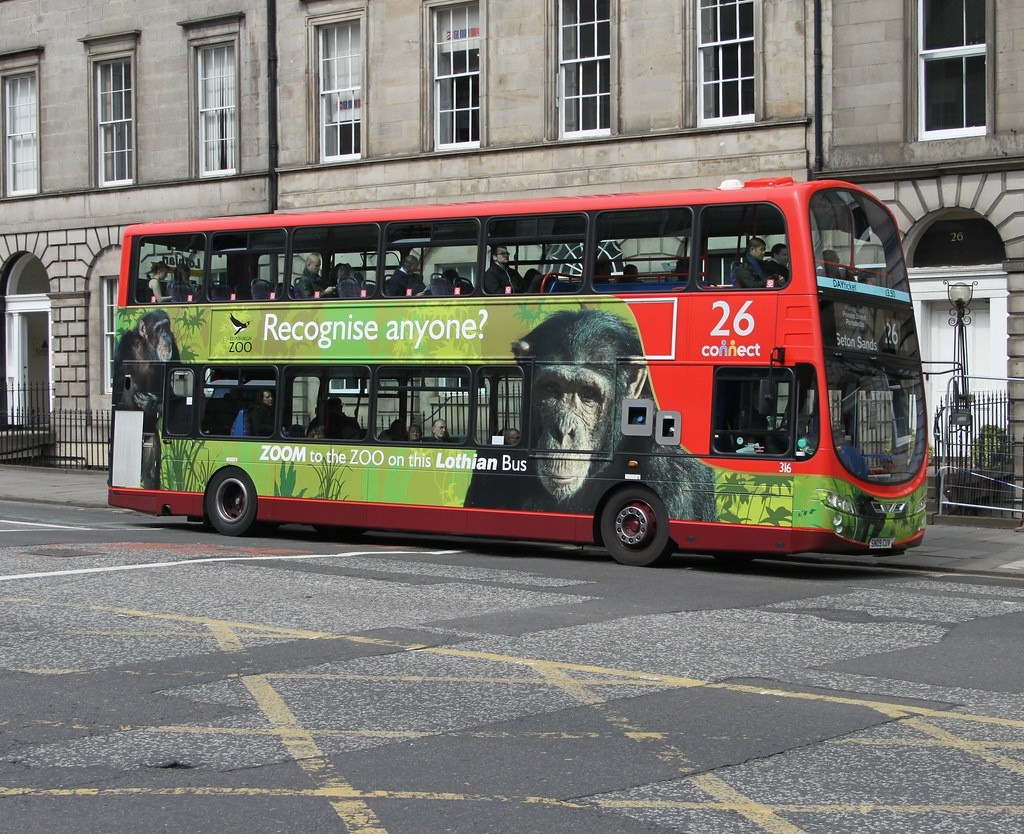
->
[{"xmin": 107, "ymin": 177, "xmax": 929, "ymax": 569}]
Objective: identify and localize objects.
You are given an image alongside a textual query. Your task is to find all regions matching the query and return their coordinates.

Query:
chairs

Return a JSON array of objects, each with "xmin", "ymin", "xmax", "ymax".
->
[
  {"xmin": 377, "ymin": 429, "xmax": 394, "ymax": 441},
  {"xmin": 287, "ymin": 424, "xmax": 306, "ymax": 438},
  {"xmin": 168, "ymin": 272, "xmax": 475, "ymax": 300},
  {"xmin": 729, "ymin": 260, "xmax": 741, "ymax": 286}
]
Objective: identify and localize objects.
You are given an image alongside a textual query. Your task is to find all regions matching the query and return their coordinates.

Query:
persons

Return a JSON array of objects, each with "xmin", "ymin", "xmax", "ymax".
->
[
  {"xmin": 388, "ymin": 254, "xmax": 428, "ymax": 296},
  {"xmin": 298, "ymin": 254, "xmax": 337, "ymax": 299},
  {"xmin": 816, "ymin": 250, "xmax": 856, "ymax": 282},
  {"xmin": 593, "ymin": 260, "xmax": 614, "ymax": 284},
  {"xmin": 307, "ymin": 397, "xmax": 347, "ymax": 438},
  {"xmin": 249, "ymin": 389, "xmax": 276, "ymax": 436},
  {"xmin": 441, "ymin": 269, "xmax": 474, "ymax": 294},
  {"xmin": 334, "ymin": 263, "xmax": 354, "ymax": 289},
  {"xmin": 408, "ymin": 425, "xmax": 422, "ymax": 442},
  {"xmin": 734, "ymin": 237, "xmax": 791, "ymax": 288},
  {"xmin": 431, "ymin": 419, "xmax": 447, "ymax": 442},
  {"xmin": 378, "ymin": 420, "xmax": 405, "ymax": 441},
  {"xmin": 500, "ymin": 428, "xmax": 520, "ymax": 445},
  {"xmin": 144, "ymin": 261, "xmax": 191, "ymax": 302},
  {"xmin": 485, "ymin": 244, "xmax": 526, "ymax": 294},
  {"xmin": 675, "ymin": 258, "xmax": 689, "ymax": 280},
  {"xmin": 617, "ymin": 264, "xmax": 642, "ymax": 283}
]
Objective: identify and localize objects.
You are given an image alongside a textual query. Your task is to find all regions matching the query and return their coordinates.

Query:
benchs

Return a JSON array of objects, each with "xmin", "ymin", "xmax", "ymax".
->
[
  {"xmin": 135, "ymin": 278, "xmax": 170, "ymax": 303},
  {"xmin": 230, "ymin": 409, "xmax": 251, "ymax": 436}
]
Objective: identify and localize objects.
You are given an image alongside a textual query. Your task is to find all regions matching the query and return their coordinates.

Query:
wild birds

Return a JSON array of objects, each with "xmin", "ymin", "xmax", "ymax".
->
[{"xmin": 230, "ymin": 313, "xmax": 251, "ymax": 334}]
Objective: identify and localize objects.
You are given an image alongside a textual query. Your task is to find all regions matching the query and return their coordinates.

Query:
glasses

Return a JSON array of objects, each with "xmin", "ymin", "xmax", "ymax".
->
[{"xmin": 497, "ymin": 252, "xmax": 510, "ymax": 256}]
